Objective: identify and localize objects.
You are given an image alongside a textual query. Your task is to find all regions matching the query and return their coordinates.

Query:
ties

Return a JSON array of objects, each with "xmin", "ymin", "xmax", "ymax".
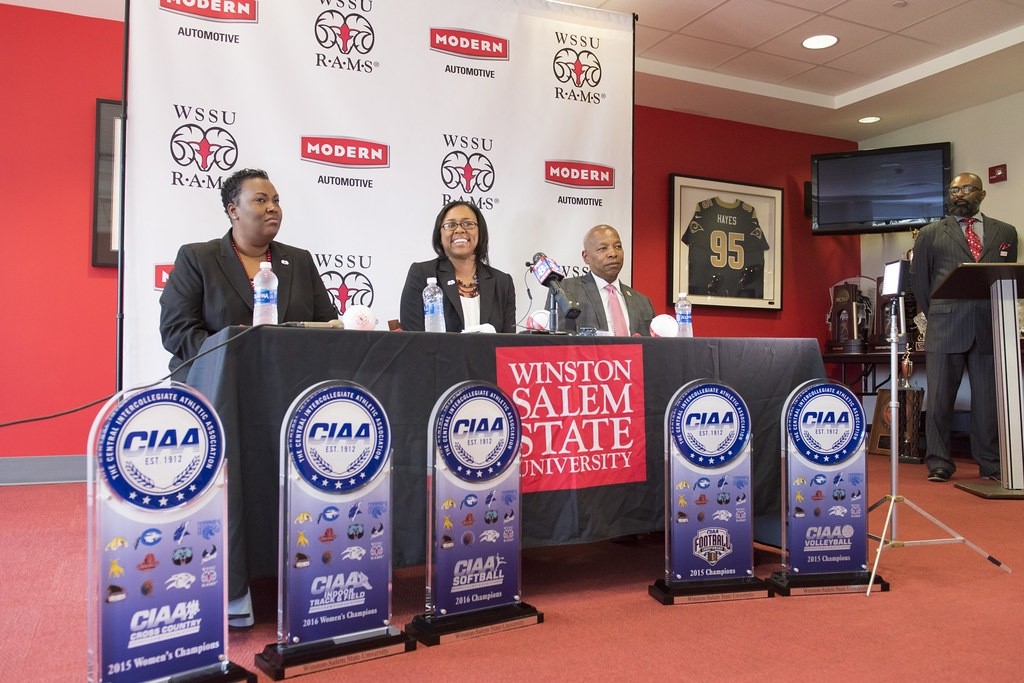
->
[
  {"xmin": 605, "ymin": 285, "xmax": 628, "ymax": 336},
  {"xmin": 958, "ymin": 218, "xmax": 983, "ymax": 262}
]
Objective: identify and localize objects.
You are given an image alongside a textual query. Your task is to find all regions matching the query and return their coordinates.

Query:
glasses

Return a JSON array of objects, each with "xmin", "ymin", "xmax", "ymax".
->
[
  {"xmin": 439, "ymin": 221, "xmax": 478, "ymax": 231},
  {"xmin": 948, "ymin": 186, "xmax": 981, "ymax": 196}
]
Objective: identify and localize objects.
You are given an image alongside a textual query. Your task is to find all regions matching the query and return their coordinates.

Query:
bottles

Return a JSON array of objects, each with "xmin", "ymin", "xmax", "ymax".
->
[
  {"xmin": 422, "ymin": 277, "xmax": 445, "ymax": 333},
  {"xmin": 674, "ymin": 293, "xmax": 694, "ymax": 338},
  {"xmin": 253, "ymin": 262, "xmax": 278, "ymax": 328}
]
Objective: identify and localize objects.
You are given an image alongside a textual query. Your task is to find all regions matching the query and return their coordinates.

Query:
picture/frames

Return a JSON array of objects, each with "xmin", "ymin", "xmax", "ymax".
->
[
  {"xmin": 91, "ymin": 97, "xmax": 124, "ymax": 267},
  {"xmin": 668, "ymin": 173, "xmax": 784, "ymax": 310}
]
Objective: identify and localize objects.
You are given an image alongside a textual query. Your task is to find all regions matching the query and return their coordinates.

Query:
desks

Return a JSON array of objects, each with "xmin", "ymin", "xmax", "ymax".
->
[
  {"xmin": 186, "ymin": 324, "xmax": 822, "ymax": 592},
  {"xmin": 822, "ymin": 351, "xmax": 928, "ymax": 433}
]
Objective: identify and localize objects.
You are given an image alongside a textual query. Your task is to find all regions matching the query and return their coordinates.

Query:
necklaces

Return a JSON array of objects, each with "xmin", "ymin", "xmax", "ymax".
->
[
  {"xmin": 232, "ymin": 242, "xmax": 271, "ymax": 289},
  {"xmin": 456, "ymin": 274, "xmax": 479, "ymax": 298}
]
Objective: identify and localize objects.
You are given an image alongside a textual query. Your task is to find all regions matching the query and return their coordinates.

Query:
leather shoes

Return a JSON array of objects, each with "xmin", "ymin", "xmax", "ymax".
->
[
  {"xmin": 928, "ymin": 467, "xmax": 949, "ymax": 481},
  {"xmin": 984, "ymin": 471, "xmax": 1001, "ymax": 482}
]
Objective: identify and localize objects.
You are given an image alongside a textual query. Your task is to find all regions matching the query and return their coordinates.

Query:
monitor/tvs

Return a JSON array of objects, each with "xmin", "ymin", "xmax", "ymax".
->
[{"xmin": 811, "ymin": 142, "xmax": 951, "ymax": 236}]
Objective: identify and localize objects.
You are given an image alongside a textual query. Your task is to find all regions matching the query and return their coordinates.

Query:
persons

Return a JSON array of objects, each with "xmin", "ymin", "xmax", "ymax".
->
[
  {"xmin": 911, "ymin": 173, "xmax": 1020, "ymax": 482},
  {"xmin": 545, "ymin": 224, "xmax": 655, "ymax": 337},
  {"xmin": 160, "ymin": 170, "xmax": 338, "ymax": 386},
  {"xmin": 400, "ymin": 201, "xmax": 516, "ymax": 333}
]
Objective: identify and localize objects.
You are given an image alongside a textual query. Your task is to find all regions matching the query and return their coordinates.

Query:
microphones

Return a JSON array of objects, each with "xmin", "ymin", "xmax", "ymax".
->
[
  {"xmin": 282, "ymin": 319, "xmax": 345, "ymax": 329},
  {"xmin": 525, "ymin": 252, "xmax": 582, "ymax": 319}
]
